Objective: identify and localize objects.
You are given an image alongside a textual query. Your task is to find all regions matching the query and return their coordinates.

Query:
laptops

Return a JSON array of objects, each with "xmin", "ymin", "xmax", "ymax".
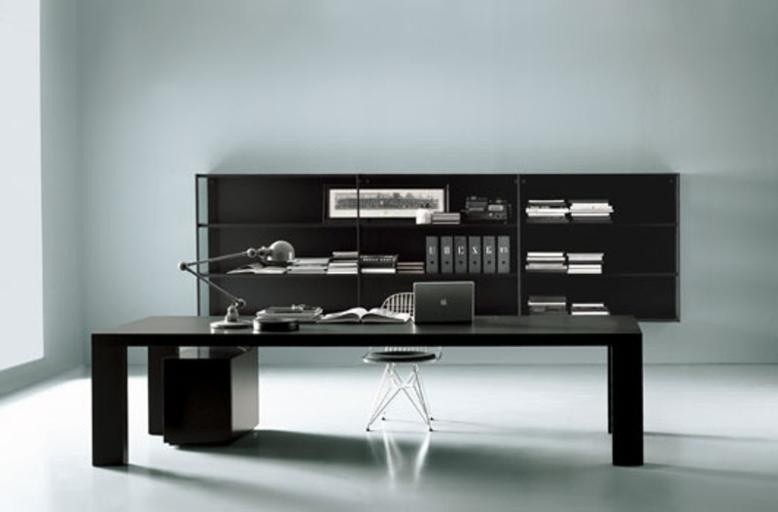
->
[{"xmin": 411, "ymin": 281, "xmax": 474, "ymax": 325}]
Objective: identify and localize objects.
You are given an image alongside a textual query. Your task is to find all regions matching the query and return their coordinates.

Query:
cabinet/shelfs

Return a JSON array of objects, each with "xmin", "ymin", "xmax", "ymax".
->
[{"xmin": 195, "ymin": 174, "xmax": 682, "ymax": 323}]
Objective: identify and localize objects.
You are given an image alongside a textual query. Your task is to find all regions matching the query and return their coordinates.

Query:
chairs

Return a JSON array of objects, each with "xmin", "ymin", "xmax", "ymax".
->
[{"xmin": 362, "ymin": 293, "xmax": 442, "ymax": 432}]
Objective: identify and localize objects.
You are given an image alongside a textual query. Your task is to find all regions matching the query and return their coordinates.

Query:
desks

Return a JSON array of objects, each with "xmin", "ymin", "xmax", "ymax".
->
[{"xmin": 90, "ymin": 317, "xmax": 644, "ymax": 467}]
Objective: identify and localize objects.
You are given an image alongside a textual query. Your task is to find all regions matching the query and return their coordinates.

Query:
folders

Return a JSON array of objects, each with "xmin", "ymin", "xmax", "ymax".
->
[
  {"xmin": 440, "ymin": 236, "xmax": 453, "ymax": 274},
  {"xmin": 497, "ymin": 236, "xmax": 510, "ymax": 274},
  {"xmin": 468, "ymin": 236, "xmax": 481, "ymax": 273},
  {"xmin": 483, "ymin": 236, "xmax": 496, "ymax": 274},
  {"xmin": 454, "ymin": 236, "xmax": 467, "ymax": 274},
  {"xmin": 425, "ymin": 235, "xmax": 438, "ymax": 274}
]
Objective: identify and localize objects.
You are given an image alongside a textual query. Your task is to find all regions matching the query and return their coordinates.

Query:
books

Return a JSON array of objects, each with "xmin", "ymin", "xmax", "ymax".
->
[
  {"xmin": 525, "ymin": 198, "xmax": 615, "ymax": 221},
  {"xmin": 360, "ymin": 263, "xmax": 396, "ymax": 274},
  {"xmin": 226, "ymin": 261, "xmax": 286, "ymax": 275},
  {"xmin": 320, "ymin": 307, "xmax": 410, "ymax": 324},
  {"xmin": 285, "ymin": 250, "xmax": 359, "ymax": 275},
  {"xmin": 525, "ymin": 251, "xmax": 605, "ymax": 277},
  {"xmin": 257, "ymin": 306, "xmax": 323, "ymax": 323},
  {"xmin": 527, "ymin": 294, "xmax": 611, "ymax": 317},
  {"xmin": 397, "ymin": 262, "xmax": 425, "ymax": 274},
  {"xmin": 431, "ymin": 212, "xmax": 460, "ymax": 224}
]
